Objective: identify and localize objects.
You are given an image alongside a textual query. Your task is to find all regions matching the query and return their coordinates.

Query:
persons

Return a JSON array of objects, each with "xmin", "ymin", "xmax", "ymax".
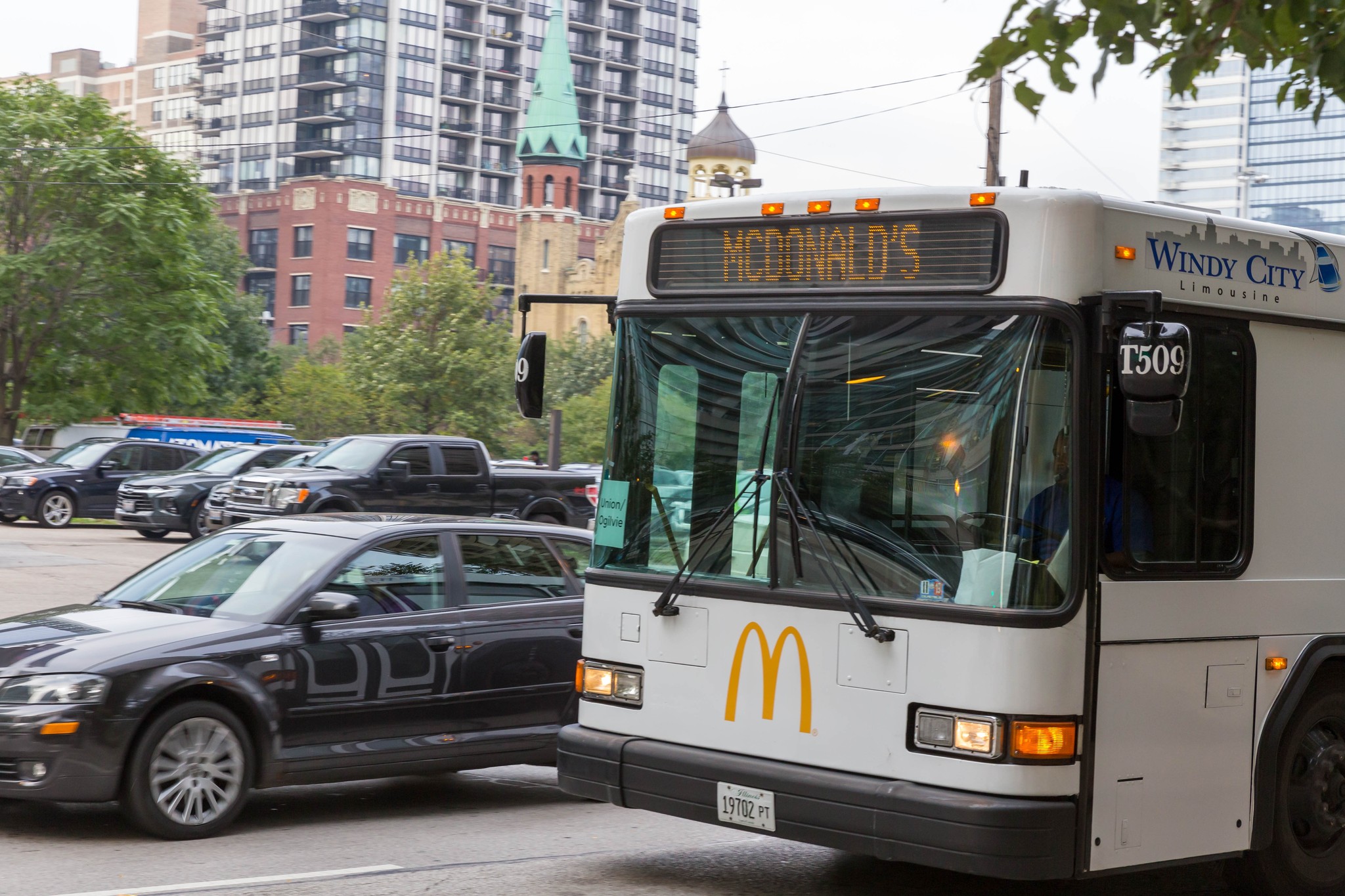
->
[
  {"xmin": 1016, "ymin": 425, "xmax": 1128, "ymax": 566},
  {"xmin": 531, "ymin": 452, "xmax": 543, "ymax": 470}
]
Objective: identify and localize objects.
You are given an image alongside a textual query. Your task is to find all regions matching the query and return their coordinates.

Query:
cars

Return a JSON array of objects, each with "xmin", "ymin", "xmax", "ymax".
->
[
  {"xmin": 1, "ymin": 445, "xmax": 45, "ymax": 467},
  {"xmin": 0, "ymin": 435, "xmax": 207, "ymax": 529},
  {"xmin": 0, "ymin": 511, "xmax": 599, "ymax": 839},
  {"xmin": 495, "ymin": 457, "xmax": 778, "ymax": 535},
  {"xmin": 115, "ymin": 444, "xmax": 326, "ymax": 544}
]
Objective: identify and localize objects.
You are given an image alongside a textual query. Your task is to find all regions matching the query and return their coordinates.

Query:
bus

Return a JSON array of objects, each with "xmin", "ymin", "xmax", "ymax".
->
[{"xmin": 512, "ymin": 182, "xmax": 1345, "ymax": 896}]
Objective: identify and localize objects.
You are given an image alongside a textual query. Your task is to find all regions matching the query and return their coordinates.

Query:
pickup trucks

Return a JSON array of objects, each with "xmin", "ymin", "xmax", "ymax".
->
[{"xmin": 202, "ymin": 432, "xmax": 600, "ymax": 529}]
